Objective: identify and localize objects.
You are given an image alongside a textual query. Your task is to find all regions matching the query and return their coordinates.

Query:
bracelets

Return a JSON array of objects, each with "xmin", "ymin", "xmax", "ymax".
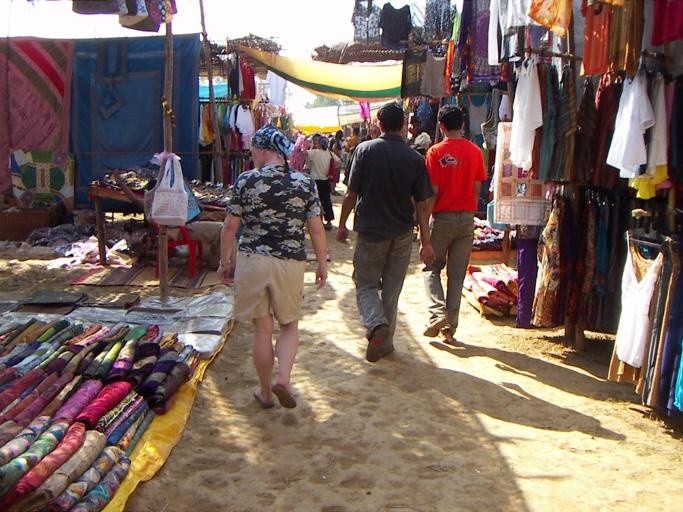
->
[{"xmin": 219, "ymin": 259, "xmax": 231, "ymax": 267}]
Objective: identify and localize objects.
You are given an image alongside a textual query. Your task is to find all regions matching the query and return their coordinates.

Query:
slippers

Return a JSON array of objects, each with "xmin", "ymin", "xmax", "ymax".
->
[
  {"xmin": 272, "ymin": 383, "xmax": 297, "ymax": 409},
  {"xmin": 253, "ymin": 386, "xmax": 275, "ymax": 409}
]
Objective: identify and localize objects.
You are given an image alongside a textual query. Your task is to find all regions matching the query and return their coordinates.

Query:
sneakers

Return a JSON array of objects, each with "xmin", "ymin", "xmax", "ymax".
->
[
  {"xmin": 366, "ymin": 325, "xmax": 395, "ymax": 362},
  {"xmin": 324, "ymin": 223, "xmax": 333, "ymax": 229},
  {"xmin": 423, "ymin": 317, "xmax": 457, "ymax": 346}
]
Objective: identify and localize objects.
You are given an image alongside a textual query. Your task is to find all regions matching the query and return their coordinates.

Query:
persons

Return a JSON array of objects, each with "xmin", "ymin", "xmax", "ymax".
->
[
  {"xmin": 416, "ymin": 103, "xmax": 488, "ymax": 343},
  {"xmin": 215, "ymin": 124, "xmax": 328, "ymax": 409},
  {"xmin": 334, "ymin": 102, "xmax": 437, "ymax": 364},
  {"xmin": 294, "ymin": 117, "xmax": 433, "ymax": 230},
  {"xmin": 143, "ymin": 179, "xmax": 238, "ymax": 276}
]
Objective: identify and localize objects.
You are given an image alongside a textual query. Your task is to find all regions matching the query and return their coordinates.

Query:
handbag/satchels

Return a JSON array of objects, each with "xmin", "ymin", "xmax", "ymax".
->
[
  {"xmin": 143, "ymin": 155, "xmax": 203, "ymax": 230},
  {"xmin": 326, "ymin": 150, "xmax": 338, "ymax": 183}
]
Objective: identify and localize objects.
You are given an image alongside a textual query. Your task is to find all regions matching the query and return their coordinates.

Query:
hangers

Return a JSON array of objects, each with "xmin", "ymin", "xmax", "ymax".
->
[
  {"xmin": 622, "ymin": 207, "xmax": 682, "ymax": 254},
  {"xmin": 522, "ymin": 47, "xmax": 534, "ymax": 68}
]
